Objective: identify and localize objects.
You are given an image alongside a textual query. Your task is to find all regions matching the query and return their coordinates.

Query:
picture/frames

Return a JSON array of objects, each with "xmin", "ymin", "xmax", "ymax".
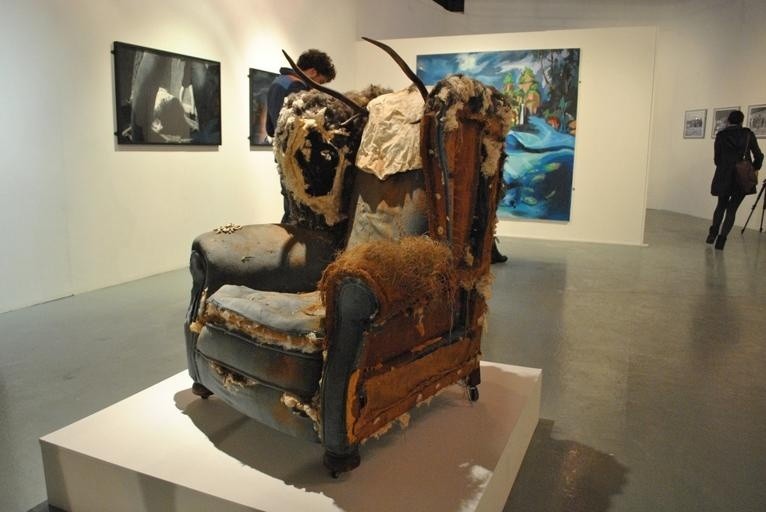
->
[
  {"xmin": 683, "ymin": 108, "xmax": 707, "ymax": 139},
  {"xmin": 113, "ymin": 41, "xmax": 222, "ymax": 147},
  {"xmin": 710, "ymin": 106, "xmax": 741, "ymax": 139},
  {"xmin": 249, "ymin": 67, "xmax": 280, "ymax": 146},
  {"xmin": 747, "ymin": 103, "xmax": 766, "ymax": 139}
]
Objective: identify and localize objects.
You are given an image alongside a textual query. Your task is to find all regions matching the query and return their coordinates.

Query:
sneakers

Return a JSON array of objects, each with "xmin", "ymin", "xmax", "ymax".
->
[
  {"xmin": 490, "ymin": 252, "xmax": 508, "ymax": 263},
  {"xmin": 713, "ymin": 240, "xmax": 726, "ymax": 251},
  {"xmin": 705, "ymin": 232, "xmax": 716, "ymax": 244}
]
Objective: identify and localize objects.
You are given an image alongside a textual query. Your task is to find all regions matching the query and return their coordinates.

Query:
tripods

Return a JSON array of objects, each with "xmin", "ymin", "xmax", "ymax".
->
[{"xmin": 740, "ymin": 178, "xmax": 766, "ymax": 235}]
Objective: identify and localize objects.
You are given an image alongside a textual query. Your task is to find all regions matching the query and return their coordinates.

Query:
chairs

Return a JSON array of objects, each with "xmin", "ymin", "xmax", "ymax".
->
[{"xmin": 183, "ymin": 70, "xmax": 511, "ymax": 479}]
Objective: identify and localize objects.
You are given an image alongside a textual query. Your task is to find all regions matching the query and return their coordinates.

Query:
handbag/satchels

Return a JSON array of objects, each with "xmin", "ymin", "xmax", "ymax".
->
[{"xmin": 734, "ymin": 158, "xmax": 757, "ymax": 195}]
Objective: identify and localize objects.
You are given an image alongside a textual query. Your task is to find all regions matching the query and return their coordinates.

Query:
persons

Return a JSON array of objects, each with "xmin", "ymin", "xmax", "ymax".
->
[
  {"xmin": 705, "ymin": 109, "xmax": 765, "ymax": 250},
  {"xmin": 264, "ymin": 47, "xmax": 337, "ymax": 136}
]
